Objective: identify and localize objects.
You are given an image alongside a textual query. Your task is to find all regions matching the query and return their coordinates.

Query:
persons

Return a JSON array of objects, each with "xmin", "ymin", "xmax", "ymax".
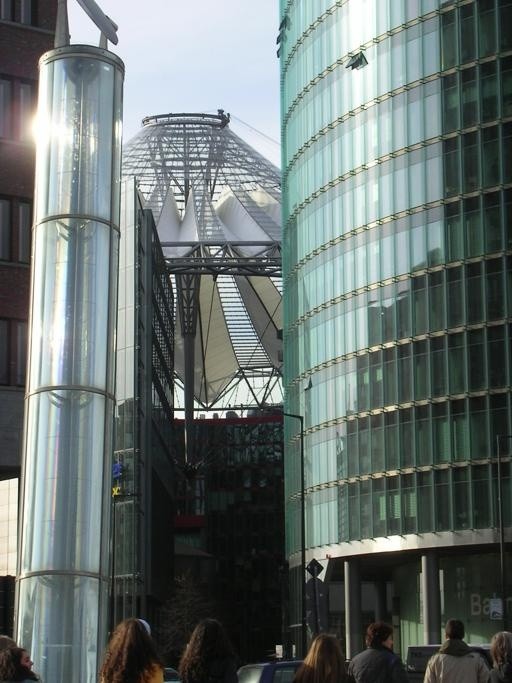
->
[
  {"xmin": 292, "ymin": 631, "xmax": 358, "ymax": 682},
  {"xmin": 0, "ymin": 634, "xmax": 17, "ymax": 650},
  {"xmin": 488, "ymin": 631, "xmax": 512, "ymax": 682},
  {"xmin": 96, "ymin": 617, "xmax": 165, "ymax": 681},
  {"xmin": 0, "ymin": 647, "xmax": 40, "ymax": 683},
  {"xmin": 346, "ymin": 621, "xmax": 409, "ymax": 682},
  {"xmin": 424, "ymin": 618, "xmax": 493, "ymax": 683},
  {"xmin": 177, "ymin": 617, "xmax": 241, "ymax": 682}
]
